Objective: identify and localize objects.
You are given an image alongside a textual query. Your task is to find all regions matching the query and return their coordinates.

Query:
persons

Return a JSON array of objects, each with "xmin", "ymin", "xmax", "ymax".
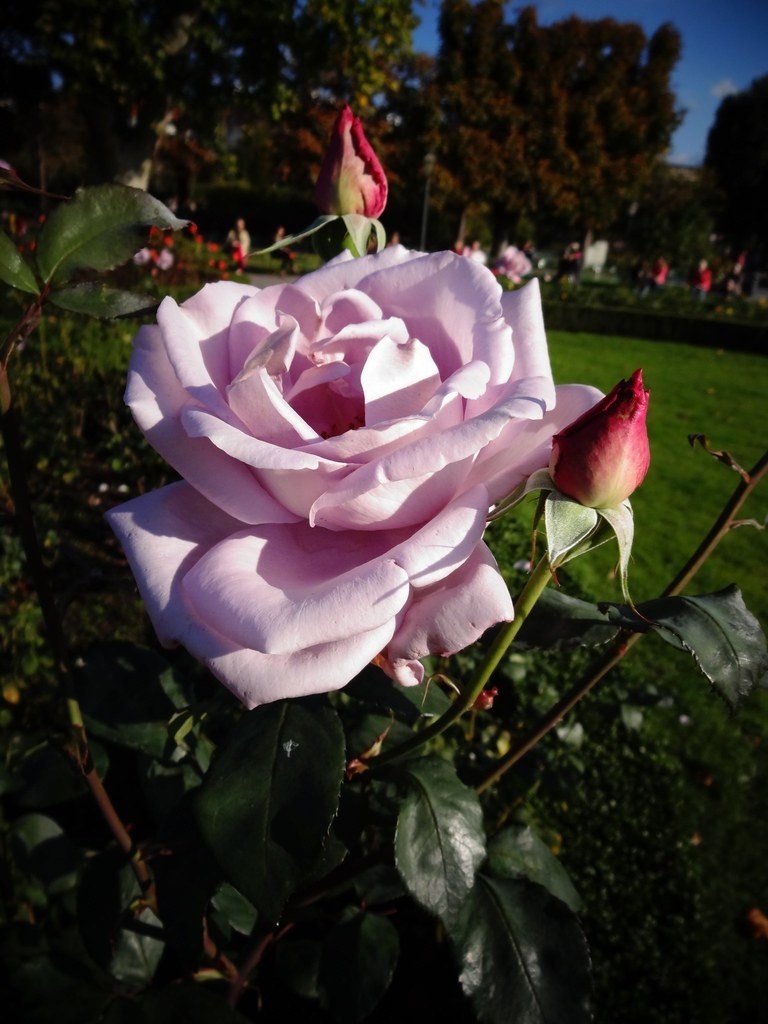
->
[
  {"xmin": 635, "ymin": 249, "xmax": 746, "ymax": 304},
  {"xmin": 223, "ymin": 214, "xmax": 294, "ymax": 273},
  {"xmin": 379, "ymin": 226, "xmax": 582, "ymax": 282}
]
